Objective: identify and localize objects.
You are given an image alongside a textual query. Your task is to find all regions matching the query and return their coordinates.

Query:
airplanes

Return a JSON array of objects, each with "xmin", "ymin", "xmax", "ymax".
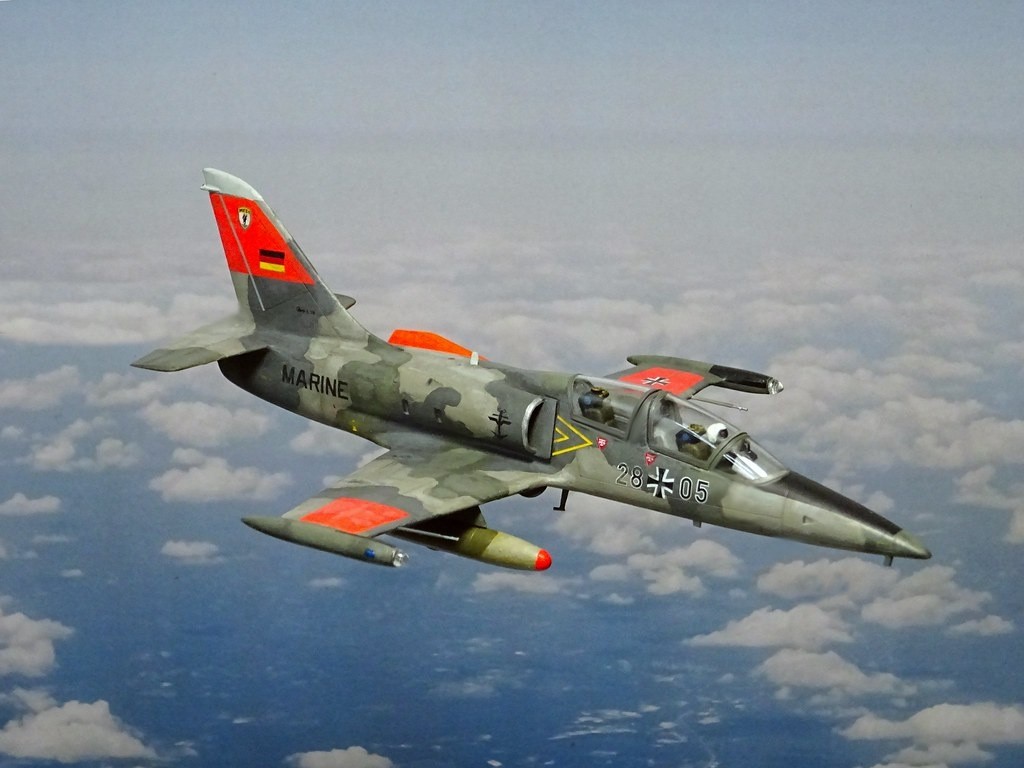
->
[{"xmin": 131, "ymin": 165, "xmax": 936, "ymax": 576}]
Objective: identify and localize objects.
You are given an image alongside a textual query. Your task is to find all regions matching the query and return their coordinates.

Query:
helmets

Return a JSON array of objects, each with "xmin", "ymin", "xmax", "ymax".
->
[{"xmin": 707, "ymin": 422, "xmax": 729, "ymax": 444}]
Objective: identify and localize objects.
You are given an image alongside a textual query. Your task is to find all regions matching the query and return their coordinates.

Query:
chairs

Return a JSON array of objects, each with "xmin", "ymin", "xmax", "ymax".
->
[
  {"xmin": 679, "ymin": 431, "xmax": 710, "ymax": 458},
  {"xmin": 586, "ymin": 404, "xmax": 614, "ymax": 426}
]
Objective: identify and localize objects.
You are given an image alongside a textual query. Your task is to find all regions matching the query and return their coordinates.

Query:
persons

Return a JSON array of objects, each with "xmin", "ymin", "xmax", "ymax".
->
[{"xmin": 706, "ymin": 423, "xmax": 736, "ymax": 462}]
